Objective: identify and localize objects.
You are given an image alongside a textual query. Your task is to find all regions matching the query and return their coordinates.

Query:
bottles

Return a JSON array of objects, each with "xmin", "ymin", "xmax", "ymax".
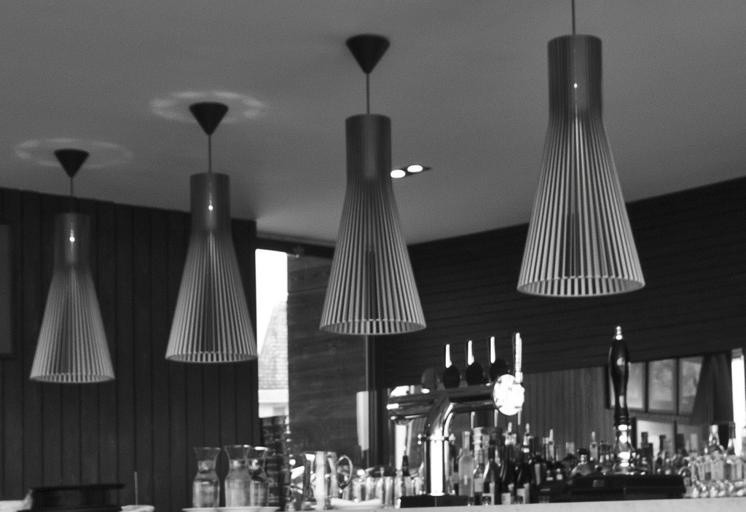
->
[
  {"xmin": 356, "ymin": 466, "xmax": 426, "ymax": 505},
  {"xmin": 190, "ymin": 439, "xmax": 268, "ymax": 507},
  {"xmin": 446, "ymin": 411, "xmax": 746, "ymax": 496}
]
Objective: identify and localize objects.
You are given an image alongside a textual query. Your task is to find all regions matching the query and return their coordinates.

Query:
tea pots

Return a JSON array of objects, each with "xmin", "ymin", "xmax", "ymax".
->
[{"xmin": 301, "ymin": 447, "xmax": 353, "ymax": 506}]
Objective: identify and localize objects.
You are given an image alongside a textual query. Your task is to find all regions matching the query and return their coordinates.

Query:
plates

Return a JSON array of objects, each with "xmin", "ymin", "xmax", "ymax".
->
[{"xmin": 180, "ymin": 506, "xmax": 282, "ymax": 511}]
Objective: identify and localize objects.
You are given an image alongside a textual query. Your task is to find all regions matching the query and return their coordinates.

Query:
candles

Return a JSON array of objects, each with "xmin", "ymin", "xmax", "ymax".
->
[{"xmin": 443, "ymin": 336, "xmax": 496, "ymax": 369}]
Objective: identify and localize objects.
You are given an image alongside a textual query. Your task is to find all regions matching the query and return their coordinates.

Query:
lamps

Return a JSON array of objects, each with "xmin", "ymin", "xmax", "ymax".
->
[
  {"xmin": 318, "ymin": 33, "xmax": 427, "ymax": 339},
  {"xmin": 513, "ymin": 0, "xmax": 646, "ymax": 300},
  {"xmin": 28, "ymin": 147, "xmax": 117, "ymax": 384},
  {"xmin": 166, "ymin": 102, "xmax": 259, "ymax": 365}
]
